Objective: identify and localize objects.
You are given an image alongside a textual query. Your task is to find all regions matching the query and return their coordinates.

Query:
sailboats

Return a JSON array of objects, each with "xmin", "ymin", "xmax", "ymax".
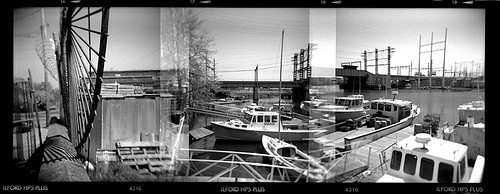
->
[{"xmin": 261, "ymin": 30, "xmax": 328, "ymax": 183}]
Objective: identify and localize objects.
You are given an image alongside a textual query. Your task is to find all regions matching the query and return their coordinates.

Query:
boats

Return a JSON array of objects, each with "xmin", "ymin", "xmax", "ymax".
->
[
  {"xmin": 310, "ymin": 88, "xmax": 370, "ymax": 122},
  {"xmin": 309, "ymin": 90, "xmax": 421, "ymax": 158},
  {"xmin": 210, "ymin": 103, "xmax": 328, "ymax": 141},
  {"xmin": 375, "ymin": 94, "xmax": 485, "ymax": 183}
]
